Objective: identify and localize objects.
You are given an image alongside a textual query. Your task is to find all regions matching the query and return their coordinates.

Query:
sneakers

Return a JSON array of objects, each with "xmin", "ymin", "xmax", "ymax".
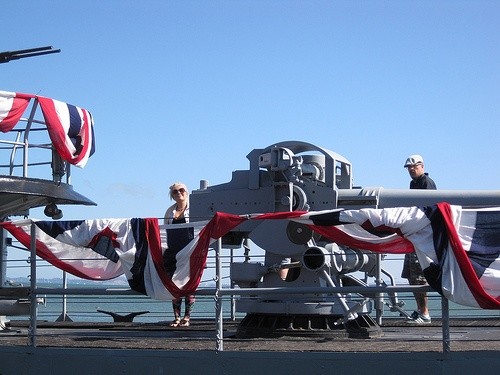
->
[
  {"xmin": 406, "ymin": 315, "xmax": 431, "ymax": 323},
  {"xmin": 407, "ymin": 310, "xmax": 423, "ymax": 319}
]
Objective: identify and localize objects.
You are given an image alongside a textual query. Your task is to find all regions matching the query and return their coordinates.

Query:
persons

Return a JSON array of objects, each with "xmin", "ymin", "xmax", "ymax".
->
[
  {"xmin": 265, "ymin": 250, "xmax": 291, "ymax": 280},
  {"xmin": 400, "ymin": 155, "xmax": 437, "ymax": 324},
  {"xmin": 164, "ymin": 183, "xmax": 196, "ymax": 326}
]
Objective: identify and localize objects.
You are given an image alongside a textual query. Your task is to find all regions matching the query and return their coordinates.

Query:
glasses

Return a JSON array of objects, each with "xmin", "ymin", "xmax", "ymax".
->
[{"xmin": 172, "ymin": 188, "xmax": 185, "ymax": 195}]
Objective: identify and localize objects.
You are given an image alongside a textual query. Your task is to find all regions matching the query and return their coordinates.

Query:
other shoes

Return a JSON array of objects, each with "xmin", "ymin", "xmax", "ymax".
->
[
  {"xmin": 179, "ymin": 318, "xmax": 190, "ymax": 326},
  {"xmin": 170, "ymin": 318, "xmax": 180, "ymax": 327}
]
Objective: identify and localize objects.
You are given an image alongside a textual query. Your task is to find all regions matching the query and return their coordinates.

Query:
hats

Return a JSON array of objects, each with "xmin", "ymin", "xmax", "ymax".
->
[{"xmin": 404, "ymin": 154, "xmax": 423, "ymax": 166}]
